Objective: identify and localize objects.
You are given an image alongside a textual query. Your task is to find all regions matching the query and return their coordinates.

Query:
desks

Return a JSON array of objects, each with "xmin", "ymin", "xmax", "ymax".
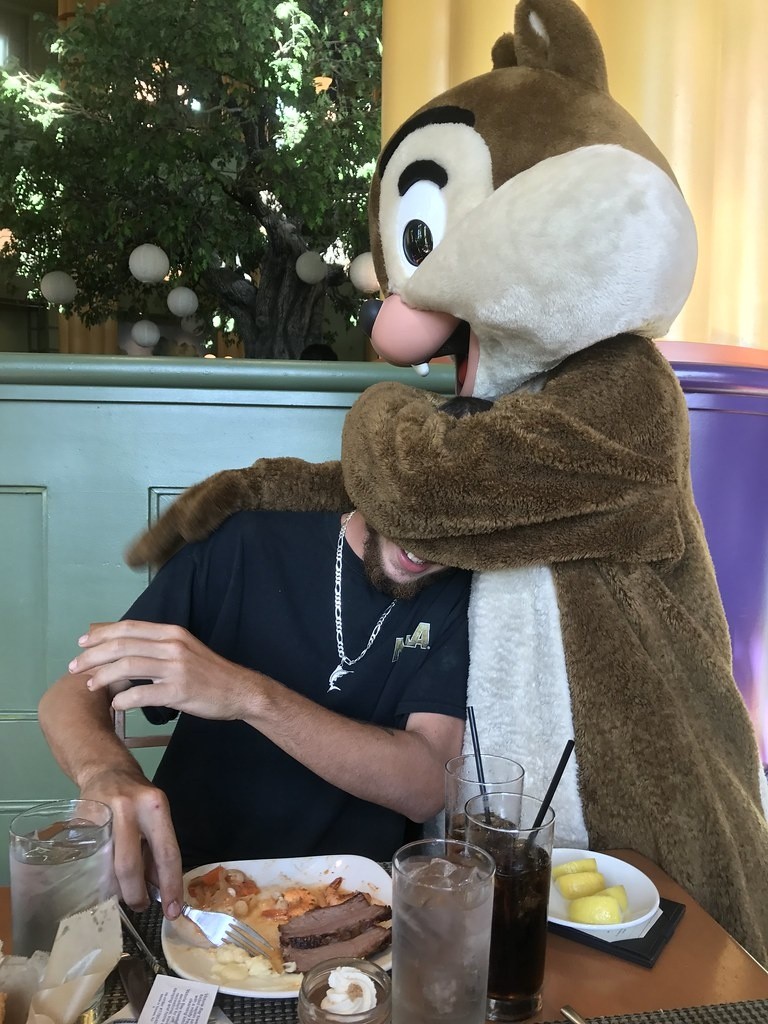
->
[{"xmin": 0, "ymin": 848, "xmax": 767, "ymax": 1024}]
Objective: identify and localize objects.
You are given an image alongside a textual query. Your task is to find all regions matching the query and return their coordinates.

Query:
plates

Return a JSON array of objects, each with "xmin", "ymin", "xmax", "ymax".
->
[
  {"xmin": 546, "ymin": 849, "xmax": 663, "ymax": 942},
  {"xmin": 160, "ymin": 853, "xmax": 395, "ymax": 999}
]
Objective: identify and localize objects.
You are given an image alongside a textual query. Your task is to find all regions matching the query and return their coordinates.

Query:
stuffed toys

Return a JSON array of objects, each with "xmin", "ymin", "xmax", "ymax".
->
[{"xmin": 126, "ymin": 0, "xmax": 768, "ymax": 971}]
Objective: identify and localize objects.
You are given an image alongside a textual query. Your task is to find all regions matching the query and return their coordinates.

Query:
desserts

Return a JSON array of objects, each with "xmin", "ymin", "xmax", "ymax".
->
[{"xmin": 321, "ymin": 968, "xmax": 377, "ymax": 1023}]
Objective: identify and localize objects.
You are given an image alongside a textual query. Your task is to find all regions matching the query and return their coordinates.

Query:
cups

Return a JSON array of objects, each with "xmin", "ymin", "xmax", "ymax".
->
[
  {"xmin": 299, "ymin": 957, "xmax": 392, "ymax": 1024},
  {"xmin": 10, "ymin": 796, "xmax": 113, "ymax": 961},
  {"xmin": 443, "ymin": 752, "xmax": 524, "ymax": 867},
  {"xmin": 466, "ymin": 792, "xmax": 555, "ymax": 1022},
  {"xmin": 391, "ymin": 839, "xmax": 495, "ymax": 1024}
]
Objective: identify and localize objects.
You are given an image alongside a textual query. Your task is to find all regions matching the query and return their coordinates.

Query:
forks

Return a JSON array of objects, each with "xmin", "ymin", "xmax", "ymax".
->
[{"xmin": 141, "ymin": 880, "xmax": 275, "ymax": 963}]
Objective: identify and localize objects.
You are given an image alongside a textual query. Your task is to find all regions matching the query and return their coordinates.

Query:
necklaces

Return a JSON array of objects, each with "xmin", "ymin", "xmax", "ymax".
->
[{"xmin": 326, "ymin": 509, "xmax": 397, "ymax": 693}]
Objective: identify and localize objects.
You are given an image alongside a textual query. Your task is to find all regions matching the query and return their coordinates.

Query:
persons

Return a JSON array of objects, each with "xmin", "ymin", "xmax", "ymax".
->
[{"xmin": 37, "ymin": 395, "xmax": 494, "ymax": 921}]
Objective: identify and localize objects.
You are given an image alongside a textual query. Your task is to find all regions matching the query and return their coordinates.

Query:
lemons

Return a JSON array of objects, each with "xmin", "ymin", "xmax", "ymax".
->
[
  {"xmin": 555, "ymin": 872, "xmax": 605, "ymax": 899},
  {"xmin": 571, "ymin": 897, "xmax": 623, "ymax": 924},
  {"xmin": 552, "ymin": 859, "xmax": 597, "ymax": 879},
  {"xmin": 592, "ymin": 885, "xmax": 626, "ymax": 911}
]
